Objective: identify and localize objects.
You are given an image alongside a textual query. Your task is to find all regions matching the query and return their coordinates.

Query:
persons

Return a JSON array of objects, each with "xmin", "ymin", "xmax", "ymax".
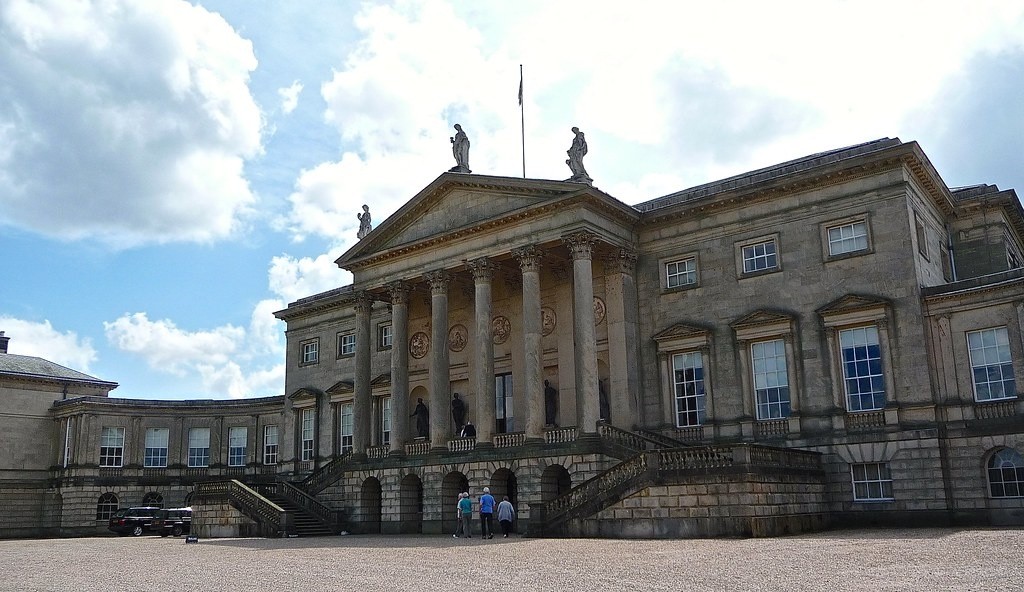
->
[
  {"xmin": 410, "ymin": 397, "xmax": 428, "ymax": 437},
  {"xmin": 453, "ymin": 491, "xmax": 472, "ymax": 538},
  {"xmin": 545, "ymin": 379, "xmax": 556, "ymax": 424},
  {"xmin": 497, "ymin": 496, "xmax": 515, "ymax": 538},
  {"xmin": 357, "ymin": 204, "xmax": 372, "ymax": 238},
  {"xmin": 479, "ymin": 487, "xmax": 495, "ymax": 539},
  {"xmin": 450, "ymin": 123, "xmax": 470, "ymax": 169},
  {"xmin": 566, "ymin": 127, "xmax": 589, "ymax": 179},
  {"xmin": 451, "ymin": 392, "xmax": 463, "ymax": 433},
  {"xmin": 461, "ymin": 421, "xmax": 476, "ymax": 436}
]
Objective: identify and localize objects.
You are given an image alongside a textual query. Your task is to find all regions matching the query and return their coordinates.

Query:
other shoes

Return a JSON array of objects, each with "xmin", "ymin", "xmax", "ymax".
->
[
  {"xmin": 463, "ymin": 535, "xmax": 467, "ymax": 540},
  {"xmin": 452, "ymin": 534, "xmax": 461, "ymax": 538},
  {"xmin": 467, "ymin": 535, "xmax": 472, "ymax": 539}
]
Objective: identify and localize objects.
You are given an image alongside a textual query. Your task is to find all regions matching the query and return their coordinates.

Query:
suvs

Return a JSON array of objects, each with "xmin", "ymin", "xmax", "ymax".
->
[
  {"xmin": 108, "ymin": 507, "xmax": 159, "ymax": 536},
  {"xmin": 151, "ymin": 508, "xmax": 191, "ymax": 537}
]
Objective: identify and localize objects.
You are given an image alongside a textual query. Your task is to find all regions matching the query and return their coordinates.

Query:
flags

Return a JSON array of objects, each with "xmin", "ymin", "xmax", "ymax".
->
[{"xmin": 518, "ymin": 76, "xmax": 523, "ymax": 106}]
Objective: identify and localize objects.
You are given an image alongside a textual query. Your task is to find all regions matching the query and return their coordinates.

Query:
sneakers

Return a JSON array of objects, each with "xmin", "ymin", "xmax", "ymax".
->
[
  {"xmin": 488, "ymin": 533, "xmax": 494, "ymax": 540},
  {"xmin": 503, "ymin": 533, "xmax": 507, "ymax": 538},
  {"xmin": 481, "ymin": 535, "xmax": 487, "ymax": 540}
]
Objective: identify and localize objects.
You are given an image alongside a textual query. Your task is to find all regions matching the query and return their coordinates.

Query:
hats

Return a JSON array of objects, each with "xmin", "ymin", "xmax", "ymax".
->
[
  {"xmin": 462, "ymin": 492, "xmax": 470, "ymax": 498},
  {"xmin": 483, "ymin": 486, "xmax": 491, "ymax": 494}
]
